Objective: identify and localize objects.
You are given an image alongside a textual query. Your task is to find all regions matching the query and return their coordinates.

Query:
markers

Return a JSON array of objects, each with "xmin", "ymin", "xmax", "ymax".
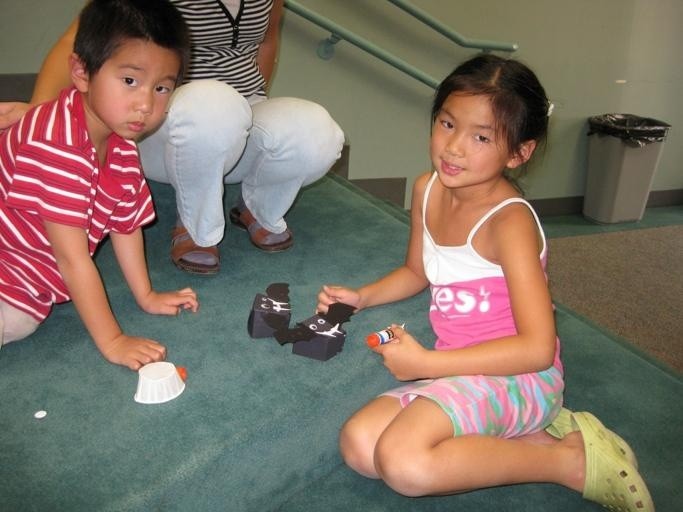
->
[{"xmin": 366, "ymin": 323, "xmax": 405, "ymax": 348}]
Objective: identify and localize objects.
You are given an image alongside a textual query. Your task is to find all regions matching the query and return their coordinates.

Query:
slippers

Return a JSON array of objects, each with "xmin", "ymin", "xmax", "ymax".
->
[
  {"xmin": 230, "ymin": 202, "xmax": 295, "ymax": 253},
  {"xmin": 544, "ymin": 407, "xmax": 639, "ymax": 472},
  {"xmin": 570, "ymin": 411, "xmax": 656, "ymax": 512},
  {"xmin": 170, "ymin": 220, "xmax": 221, "ymax": 275}
]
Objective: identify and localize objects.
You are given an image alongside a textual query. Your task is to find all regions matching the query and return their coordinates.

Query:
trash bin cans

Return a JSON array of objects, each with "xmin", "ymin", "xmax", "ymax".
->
[{"xmin": 581, "ymin": 113, "xmax": 671, "ymax": 224}]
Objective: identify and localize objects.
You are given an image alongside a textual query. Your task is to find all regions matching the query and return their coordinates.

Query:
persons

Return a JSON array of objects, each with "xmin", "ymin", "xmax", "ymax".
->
[
  {"xmin": 0, "ymin": 0, "xmax": 199, "ymax": 371},
  {"xmin": 0, "ymin": 0, "xmax": 347, "ymax": 275},
  {"xmin": 315, "ymin": 51, "xmax": 657, "ymax": 512}
]
